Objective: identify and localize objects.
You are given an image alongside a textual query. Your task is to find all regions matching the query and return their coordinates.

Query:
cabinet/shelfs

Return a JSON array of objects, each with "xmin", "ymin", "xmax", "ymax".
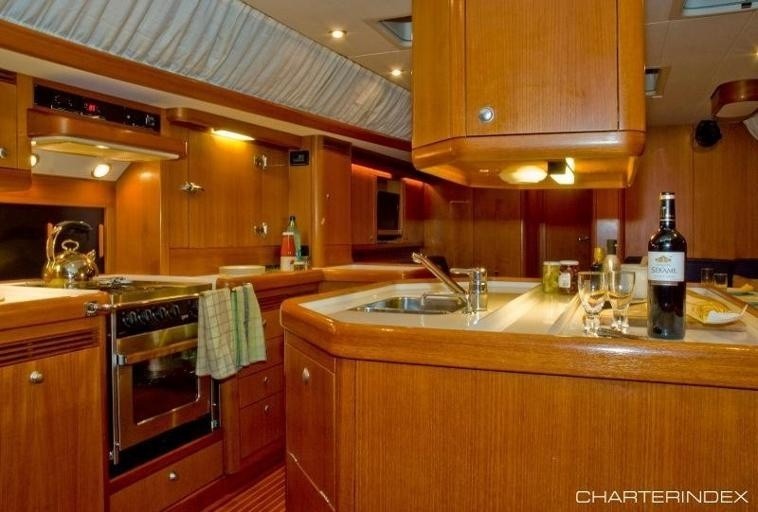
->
[
  {"xmin": 408, "ymin": 2, "xmax": 648, "ymax": 193},
  {"xmin": 277, "ymin": 332, "xmax": 337, "ymax": 512},
  {"xmin": 218, "ymin": 282, "xmax": 319, "ymax": 502},
  {"xmin": 1, "ymin": 314, "xmax": 110, "ymax": 511},
  {"xmin": 1, "ymin": 72, "xmax": 19, "ymax": 168},
  {"xmin": 109, "ymin": 426, "xmax": 223, "ymax": 511},
  {"xmin": 166, "ymin": 117, "xmax": 425, "ymax": 252},
  {"xmin": 628, "ymin": 118, "xmax": 756, "ymax": 261}
]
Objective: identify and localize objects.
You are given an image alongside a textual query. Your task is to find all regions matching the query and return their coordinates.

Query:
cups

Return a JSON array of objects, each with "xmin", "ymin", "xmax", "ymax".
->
[
  {"xmin": 713, "ymin": 273, "xmax": 728, "ymax": 292},
  {"xmin": 700, "ymin": 267, "xmax": 714, "ymax": 288}
]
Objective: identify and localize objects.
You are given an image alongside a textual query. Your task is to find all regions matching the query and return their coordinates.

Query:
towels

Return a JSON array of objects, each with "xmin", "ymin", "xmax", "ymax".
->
[
  {"xmin": 197, "ymin": 286, "xmax": 241, "ymax": 385},
  {"xmin": 232, "ymin": 284, "xmax": 269, "ymax": 367}
]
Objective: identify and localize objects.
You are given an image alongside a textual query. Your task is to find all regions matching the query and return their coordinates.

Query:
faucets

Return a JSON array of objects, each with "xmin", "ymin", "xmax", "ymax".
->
[
  {"xmin": 411, "ymin": 251, "xmax": 489, "ymax": 314},
  {"xmin": 290, "ymin": 256, "xmax": 311, "ymax": 271}
]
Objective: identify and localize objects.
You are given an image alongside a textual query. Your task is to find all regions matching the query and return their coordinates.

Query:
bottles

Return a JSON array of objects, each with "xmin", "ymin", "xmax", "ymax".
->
[
  {"xmin": 285, "ymin": 215, "xmax": 301, "ymax": 257},
  {"xmin": 601, "ymin": 239, "xmax": 622, "ymax": 290},
  {"xmin": 279, "ymin": 231, "xmax": 296, "ymax": 273},
  {"xmin": 590, "ymin": 247, "xmax": 605, "ymax": 288},
  {"xmin": 646, "ymin": 191, "xmax": 689, "ymax": 339}
]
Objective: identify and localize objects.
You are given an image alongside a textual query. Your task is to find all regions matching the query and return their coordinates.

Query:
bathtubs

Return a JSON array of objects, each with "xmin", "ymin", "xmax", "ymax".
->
[{"xmin": 310, "ymin": 261, "xmax": 426, "ymax": 281}]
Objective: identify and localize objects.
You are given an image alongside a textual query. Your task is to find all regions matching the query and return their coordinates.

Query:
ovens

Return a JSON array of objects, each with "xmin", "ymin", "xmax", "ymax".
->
[{"xmin": 106, "ymin": 320, "xmax": 219, "ymax": 485}]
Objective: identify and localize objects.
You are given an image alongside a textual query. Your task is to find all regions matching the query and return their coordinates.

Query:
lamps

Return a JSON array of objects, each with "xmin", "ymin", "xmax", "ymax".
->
[
  {"xmin": 709, "ymin": 80, "xmax": 758, "ymax": 121},
  {"xmin": 547, "ymin": 160, "xmax": 576, "ymax": 187}
]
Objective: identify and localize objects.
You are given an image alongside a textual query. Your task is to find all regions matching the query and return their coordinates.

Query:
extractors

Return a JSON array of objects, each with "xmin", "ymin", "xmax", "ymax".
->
[{"xmin": 27, "ymin": 88, "xmax": 190, "ymax": 182}]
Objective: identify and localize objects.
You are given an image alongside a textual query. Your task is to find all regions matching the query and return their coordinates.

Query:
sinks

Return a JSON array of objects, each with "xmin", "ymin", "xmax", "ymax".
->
[
  {"xmin": 366, "ymin": 296, "xmax": 467, "ymax": 312},
  {"xmin": 218, "ymin": 264, "xmax": 266, "ymax": 277},
  {"xmin": 346, "ymin": 305, "xmax": 450, "ymax": 314}
]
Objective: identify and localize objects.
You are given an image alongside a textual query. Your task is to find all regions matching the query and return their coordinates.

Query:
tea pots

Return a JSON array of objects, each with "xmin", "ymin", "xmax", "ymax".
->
[{"xmin": 43, "ymin": 219, "xmax": 100, "ymax": 286}]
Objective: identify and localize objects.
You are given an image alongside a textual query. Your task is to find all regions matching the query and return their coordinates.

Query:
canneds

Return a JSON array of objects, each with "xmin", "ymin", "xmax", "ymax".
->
[
  {"xmin": 541, "ymin": 261, "xmax": 560, "ymax": 294},
  {"xmin": 559, "ymin": 260, "xmax": 579, "ymax": 295}
]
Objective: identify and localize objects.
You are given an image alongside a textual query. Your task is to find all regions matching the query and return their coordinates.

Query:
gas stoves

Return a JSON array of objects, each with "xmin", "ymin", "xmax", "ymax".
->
[{"xmin": 16, "ymin": 276, "xmax": 213, "ymax": 339}]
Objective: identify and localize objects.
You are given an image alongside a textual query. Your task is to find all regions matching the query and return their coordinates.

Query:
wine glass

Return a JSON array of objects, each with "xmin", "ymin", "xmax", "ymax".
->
[
  {"xmin": 606, "ymin": 269, "xmax": 637, "ymax": 328},
  {"xmin": 577, "ymin": 270, "xmax": 606, "ymax": 336}
]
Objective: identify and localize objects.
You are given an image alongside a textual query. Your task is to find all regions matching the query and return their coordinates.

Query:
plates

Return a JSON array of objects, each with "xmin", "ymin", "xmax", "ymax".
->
[{"xmin": 219, "ymin": 265, "xmax": 265, "ymax": 277}]
